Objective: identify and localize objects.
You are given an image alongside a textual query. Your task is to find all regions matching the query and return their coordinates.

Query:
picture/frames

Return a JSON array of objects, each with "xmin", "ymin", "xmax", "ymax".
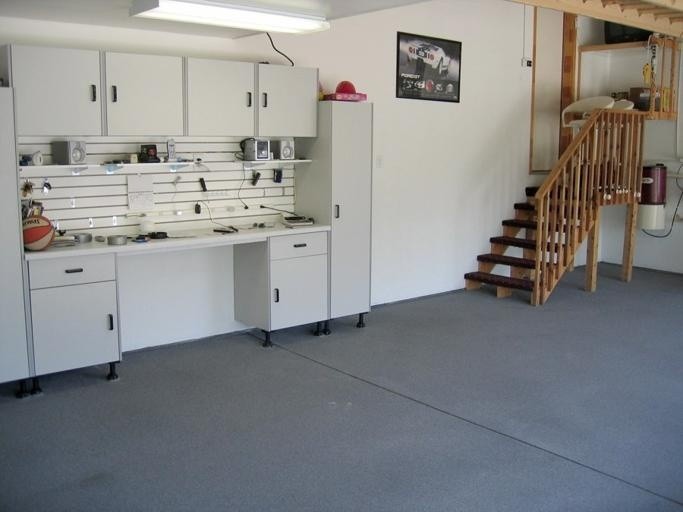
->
[{"xmin": 396, "ymin": 31, "xmax": 462, "ymax": 103}]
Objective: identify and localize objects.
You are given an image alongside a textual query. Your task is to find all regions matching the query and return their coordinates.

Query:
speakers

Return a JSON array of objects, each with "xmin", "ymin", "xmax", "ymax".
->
[
  {"xmin": 51, "ymin": 141, "xmax": 86, "ymax": 164},
  {"xmin": 277, "ymin": 139, "xmax": 295, "ymax": 159}
]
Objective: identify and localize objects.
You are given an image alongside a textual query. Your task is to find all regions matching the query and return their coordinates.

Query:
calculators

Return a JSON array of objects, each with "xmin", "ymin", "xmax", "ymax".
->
[{"xmin": 282, "ymin": 215, "xmax": 313, "ymax": 226}]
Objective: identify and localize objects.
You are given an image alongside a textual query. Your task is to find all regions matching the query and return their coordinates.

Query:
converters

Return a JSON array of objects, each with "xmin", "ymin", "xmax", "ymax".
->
[{"xmin": 194, "ymin": 206, "xmax": 201, "ymax": 215}]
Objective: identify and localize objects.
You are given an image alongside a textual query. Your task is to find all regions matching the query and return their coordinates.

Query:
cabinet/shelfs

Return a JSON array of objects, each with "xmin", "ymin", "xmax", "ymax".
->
[
  {"xmin": 1, "ymin": 44, "xmax": 184, "ymax": 136},
  {"xmin": 234, "ymin": 231, "xmax": 329, "ymax": 332},
  {"xmin": 185, "ymin": 57, "xmax": 318, "ymax": 137},
  {"xmin": 293, "ymin": 101, "xmax": 373, "ymax": 327},
  {"xmin": 0, "ymin": 87, "xmax": 30, "ymax": 384},
  {"xmin": 28, "ymin": 252, "xmax": 119, "ymax": 378}
]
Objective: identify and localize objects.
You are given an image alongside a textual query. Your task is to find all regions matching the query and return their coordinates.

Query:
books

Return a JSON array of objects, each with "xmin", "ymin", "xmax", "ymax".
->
[
  {"xmin": 49, "ymin": 234, "xmax": 76, "ymax": 247},
  {"xmin": 281, "ymin": 210, "xmax": 314, "ymax": 227}
]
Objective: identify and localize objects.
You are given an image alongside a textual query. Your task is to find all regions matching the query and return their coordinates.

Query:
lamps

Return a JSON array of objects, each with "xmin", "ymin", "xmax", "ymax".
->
[{"xmin": 129, "ymin": 0, "xmax": 331, "ymax": 34}]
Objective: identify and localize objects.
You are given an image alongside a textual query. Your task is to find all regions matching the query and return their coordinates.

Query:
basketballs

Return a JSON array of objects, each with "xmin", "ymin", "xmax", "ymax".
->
[{"xmin": 23, "ymin": 215, "xmax": 54, "ymax": 251}]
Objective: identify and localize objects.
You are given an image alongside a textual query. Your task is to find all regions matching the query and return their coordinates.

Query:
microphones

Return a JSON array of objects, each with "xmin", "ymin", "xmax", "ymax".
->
[{"xmin": 259, "ymin": 205, "xmax": 310, "ymax": 222}]
